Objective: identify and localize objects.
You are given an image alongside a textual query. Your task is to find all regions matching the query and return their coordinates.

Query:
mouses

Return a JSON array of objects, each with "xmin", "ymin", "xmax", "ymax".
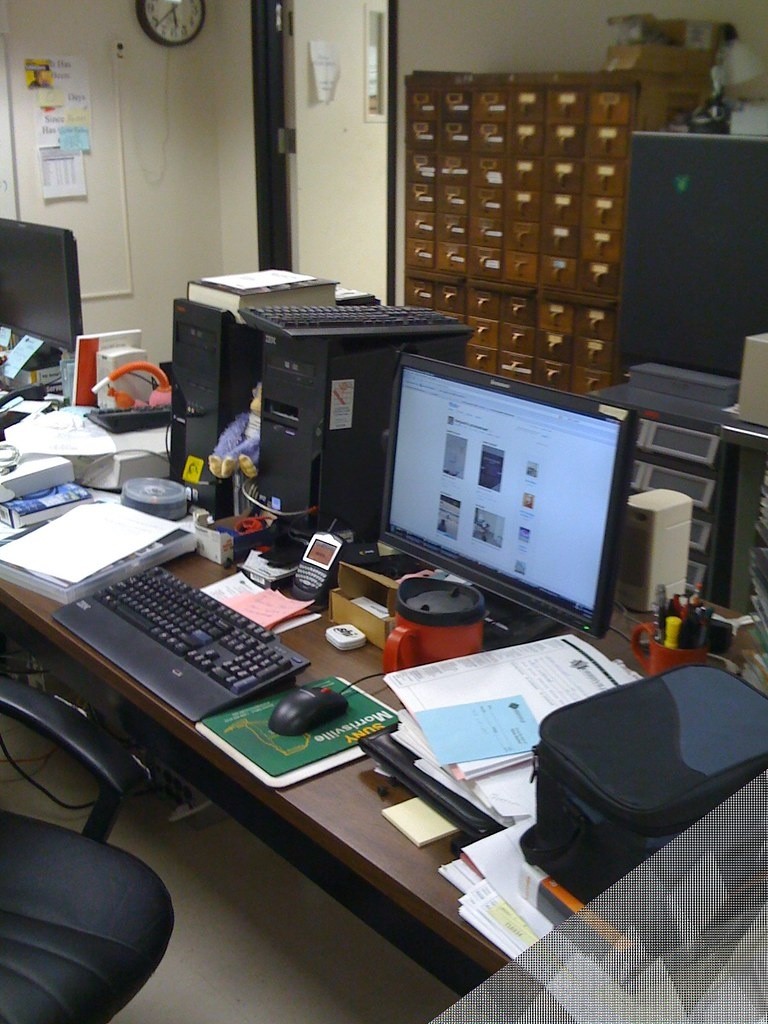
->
[{"xmin": 268, "ymin": 687, "xmax": 347, "ymax": 738}]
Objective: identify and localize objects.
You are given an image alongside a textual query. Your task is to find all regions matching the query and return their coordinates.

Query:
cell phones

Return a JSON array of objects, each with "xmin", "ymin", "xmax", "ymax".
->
[{"xmin": 293, "ymin": 517, "xmax": 346, "ymax": 593}]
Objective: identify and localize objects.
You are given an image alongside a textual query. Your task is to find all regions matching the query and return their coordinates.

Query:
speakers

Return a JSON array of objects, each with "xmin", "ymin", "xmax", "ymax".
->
[
  {"xmin": 97, "ymin": 346, "xmax": 155, "ymax": 410},
  {"xmin": 616, "ymin": 486, "xmax": 694, "ymax": 613}
]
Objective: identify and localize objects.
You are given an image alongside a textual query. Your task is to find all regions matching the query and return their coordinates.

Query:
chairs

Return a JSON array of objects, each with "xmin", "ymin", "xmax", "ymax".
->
[{"xmin": 0, "ymin": 673, "xmax": 174, "ymax": 1024}]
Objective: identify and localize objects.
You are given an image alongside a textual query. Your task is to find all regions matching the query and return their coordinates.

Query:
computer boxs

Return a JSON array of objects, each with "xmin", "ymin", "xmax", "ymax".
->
[
  {"xmin": 252, "ymin": 335, "xmax": 468, "ymax": 545},
  {"xmin": 170, "ymin": 295, "xmax": 380, "ymax": 521}
]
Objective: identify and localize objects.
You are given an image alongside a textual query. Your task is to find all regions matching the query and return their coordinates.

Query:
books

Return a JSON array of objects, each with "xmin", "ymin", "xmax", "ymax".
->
[
  {"xmin": 356, "ymin": 631, "xmax": 676, "ymax": 991},
  {"xmin": 186, "ymin": 267, "xmax": 340, "ymax": 324},
  {"xmin": 71, "ymin": 328, "xmax": 146, "ymax": 407}
]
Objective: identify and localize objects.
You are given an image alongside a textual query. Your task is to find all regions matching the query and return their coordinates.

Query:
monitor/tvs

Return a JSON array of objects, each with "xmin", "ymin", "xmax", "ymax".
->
[
  {"xmin": 0, "ymin": 218, "xmax": 83, "ymax": 371},
  {"xmin": 378, "ymin": 351, "xmax": 640, "ymax": 638}
]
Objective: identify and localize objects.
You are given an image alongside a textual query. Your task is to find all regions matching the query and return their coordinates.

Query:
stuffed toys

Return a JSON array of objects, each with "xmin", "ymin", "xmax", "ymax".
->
[{"xmin": 208, "ymin": 381, "xmax": 262, "ymax": 479}]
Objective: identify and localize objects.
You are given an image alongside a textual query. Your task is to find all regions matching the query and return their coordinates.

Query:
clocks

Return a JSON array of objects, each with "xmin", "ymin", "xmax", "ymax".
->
[{"xmin": 135, "ymin": 0, "xmax": 205, "ymax": 46}]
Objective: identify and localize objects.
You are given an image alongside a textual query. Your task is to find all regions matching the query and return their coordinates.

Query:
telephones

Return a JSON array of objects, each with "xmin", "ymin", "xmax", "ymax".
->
[{"xmin": 291, "ymin": 517, "xmax": 346, "ymax": 605}]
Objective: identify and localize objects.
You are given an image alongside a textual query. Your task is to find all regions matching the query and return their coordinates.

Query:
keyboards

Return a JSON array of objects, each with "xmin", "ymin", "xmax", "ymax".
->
[
  {"xmin": 236, "ymin": 304, "xmax": 473, "ymax": 341},
  {"xmin": 83, "ymin": 404, "xmax": 171, "ymax": 432},
  {"xmin": 52, "ymin": 566, "xmax": 311, "ymax": 722}
]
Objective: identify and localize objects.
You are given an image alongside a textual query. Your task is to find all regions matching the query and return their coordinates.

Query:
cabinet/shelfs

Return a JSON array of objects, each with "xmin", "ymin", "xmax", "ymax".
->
[
  {"xmin": 580, "ymin": 382, "xmax": 768, "ymax": 615},
  {"xmin": 404, "ymin": 68, "xmax": 715, "ymax": 395}
]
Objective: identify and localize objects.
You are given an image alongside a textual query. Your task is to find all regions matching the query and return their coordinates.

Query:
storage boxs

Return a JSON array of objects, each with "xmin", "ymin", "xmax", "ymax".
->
[
  {"xmin": 327, "ymin": 562, "xmax": 401, "ymax": 651},
  {"xmin": 196, "ymin": 509, "xmax": 277, "ymax": 565},
  {"xmin": 609, "ymin": 14, "xmax": 719, "ymax": 72}
]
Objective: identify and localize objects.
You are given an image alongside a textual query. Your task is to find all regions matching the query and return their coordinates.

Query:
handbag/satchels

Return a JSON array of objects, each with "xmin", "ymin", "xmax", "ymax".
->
[{"xmin": 519, "ymin": 662, "xmax": 768, "ymax": 901}]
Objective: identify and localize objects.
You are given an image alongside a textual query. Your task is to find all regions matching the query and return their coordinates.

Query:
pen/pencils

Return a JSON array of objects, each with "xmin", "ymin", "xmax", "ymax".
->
[{"xmin": 653, "ymin": 582, "xmax": 714, "ymax": 650}]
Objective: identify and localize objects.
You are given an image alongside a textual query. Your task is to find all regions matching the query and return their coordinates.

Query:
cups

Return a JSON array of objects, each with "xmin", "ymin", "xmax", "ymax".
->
[
  {"xmin": 630, "ymin": 621, "xmax": 708, "ymax": 676},
  {"xmin": 382, "ymin": 577, "xmax": 486, "ymax": 675}
]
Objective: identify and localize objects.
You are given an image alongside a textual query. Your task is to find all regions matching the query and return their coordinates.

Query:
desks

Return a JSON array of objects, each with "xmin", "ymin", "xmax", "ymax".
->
[{"xmin": 0, "ymin": 485, "xmax": 768, "ymax": 1024}]
